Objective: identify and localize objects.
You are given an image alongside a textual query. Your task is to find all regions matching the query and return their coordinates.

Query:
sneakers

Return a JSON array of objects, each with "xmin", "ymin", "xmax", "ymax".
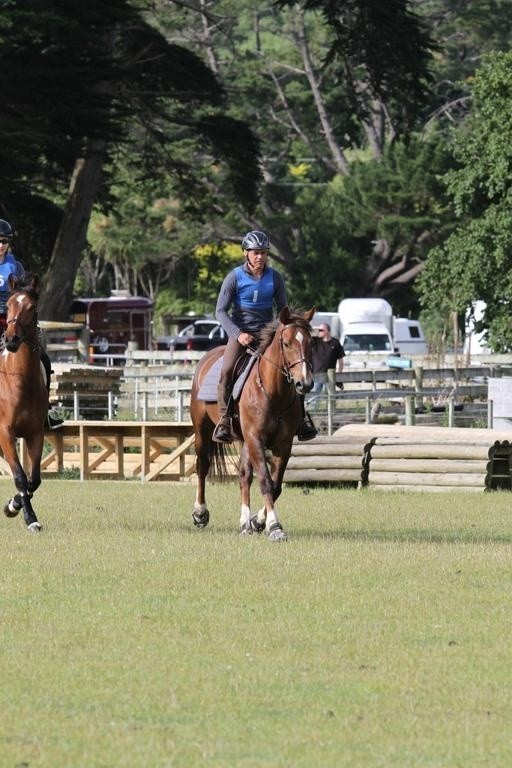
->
[
  {"xmin": 48, "ymin": 413, "xmax": 64, "ymax": 428},
  {"xmin": 216, "ymin": 424, "xmax": 231, "ymax": 442},
  {"xmin": 301, "ymin": 421, "xmax": 316, "ymax": 441}
]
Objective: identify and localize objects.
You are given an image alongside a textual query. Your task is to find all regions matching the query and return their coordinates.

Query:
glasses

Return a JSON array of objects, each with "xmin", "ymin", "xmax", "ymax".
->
[{"xmin": 0, "ymin": 240, "xmax": 10, "ymax": 245}]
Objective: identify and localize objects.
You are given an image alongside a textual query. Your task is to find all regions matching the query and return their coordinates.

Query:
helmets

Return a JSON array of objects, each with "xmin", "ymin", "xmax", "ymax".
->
[
  {"xmin": 0, "ymin": 219, "xmax": 14, "ymax": 238},
  {"xmin": 242, "ymin": 230, "xmax": 271, "ymax": 250}
]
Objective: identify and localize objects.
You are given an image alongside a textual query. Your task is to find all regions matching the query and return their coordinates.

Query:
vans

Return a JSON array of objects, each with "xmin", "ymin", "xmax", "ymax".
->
[{"xmin": 64, "ymin": 293, "xmax": 155, "ymax": 353}]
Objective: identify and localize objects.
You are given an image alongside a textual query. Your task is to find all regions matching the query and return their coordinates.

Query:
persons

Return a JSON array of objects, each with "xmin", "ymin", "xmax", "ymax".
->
[
  {"xmin": 301, "ymin": 320, "xmax": 347, "ymax": 391},
  {"xmin": 209, "ymin": 230, "xmax": 316, "ymax": 445},
  {"xmin": 1, "ymin": 218, "xmax": 56, "ymax": 379}
]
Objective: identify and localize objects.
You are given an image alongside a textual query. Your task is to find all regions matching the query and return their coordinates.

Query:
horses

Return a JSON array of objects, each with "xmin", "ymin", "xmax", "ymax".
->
[
  {"xmin": 0, "ymin": 270, "xmax": 52, "ymax": 535},
  {"xmin": 188, "ymin": 303, "xmax": 318, "ymax": 543}
]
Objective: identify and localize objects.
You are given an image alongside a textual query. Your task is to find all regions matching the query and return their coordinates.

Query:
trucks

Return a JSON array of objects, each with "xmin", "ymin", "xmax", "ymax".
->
[
  {"xmin": 308, "ymin": 297, "xmax": 431, "ymax": 369},
  {"xmin": 154, "ymin": 318, "xmax": 228, "ymax": 352}
]
